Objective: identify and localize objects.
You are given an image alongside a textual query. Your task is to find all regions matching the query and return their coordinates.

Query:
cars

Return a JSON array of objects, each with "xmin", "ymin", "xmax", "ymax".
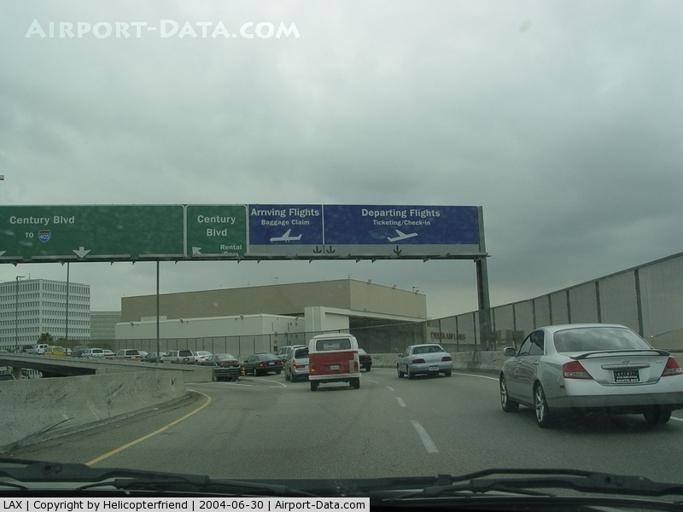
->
[
  {"xmin": 498, "ymin": 323, "xmax": 682, "ymax": 428},
  {"xmin": 358, "ymin": 348, "xmax": 372, "ymax": 372},
  {"xmin": 397, "ymin": 344, "xmax": 453, "ymax": 379},
  {"xmin": 279, "ymin": 345, "xmax": 309, "ymax": 382},
  {"xmin": 24, "ymin": 344, "xmax": 242, "ymax": 382},
  {"xmin": 243, "ymin": 353, "xmax": 283, "ymax": 377}
]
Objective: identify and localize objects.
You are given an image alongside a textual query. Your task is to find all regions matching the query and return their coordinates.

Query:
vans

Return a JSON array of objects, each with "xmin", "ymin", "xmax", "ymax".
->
[{"xmin": 308, "ymin": 333, "xmax": 360, "ymax": 390}]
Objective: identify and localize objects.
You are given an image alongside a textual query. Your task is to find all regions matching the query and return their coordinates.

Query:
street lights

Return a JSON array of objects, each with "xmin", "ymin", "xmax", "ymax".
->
[{"xmin": 16, "ymin": 276, "xmax": 25, "ymax": 353}]
